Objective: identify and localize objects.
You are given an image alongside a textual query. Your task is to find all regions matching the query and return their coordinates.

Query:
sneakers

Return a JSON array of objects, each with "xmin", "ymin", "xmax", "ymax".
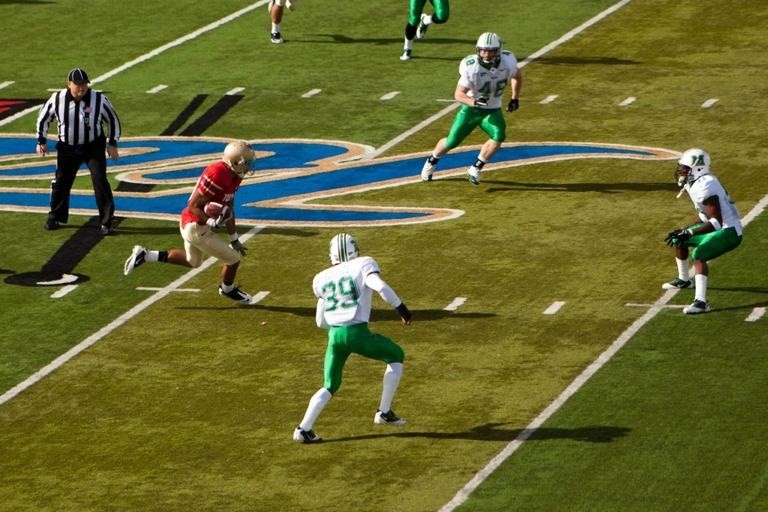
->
[
  {"xmin": 399, "ymin": 49, "xmax": 412, "ymax": 61},
  {"xmin": 466, "ymin": 165, "xmax": 480, "ymax": 187},
  {"xmin": 271, "ymin": 32, "xmax": 284, "ymax": 44},
  {"xmin": 683, "ymin": 300, "xmax": 708, "ymax": 314},
  {"xmin": 415, "ymin": 13, "xmax": 429, "ymax": 40},
  {"xmin": 373, "ymin": 409, "xmax": 406, "ymax": 425},
  {"xmin": 661, "ymin": 279, "xmax": 694, "ymax": 289},
  {"xmin": 420, "ymin": 158, "xmax": 436, "ymax": 182},
  {"xmin": 292, "ymin": 426, "xmax": 322, "ymax": 443},
  {"xmin": 98, "ymin": 220, "xmax": 113, "ymax": 235},
  {"xmin": 44, "ymin": 215, "xmax": 59, "ymax": 230},
  {"xmin": 123, "ymin": 245, "xmax": 147, "ymax": 277},
  {"xmin": 218, "ymin": 286, "xmax": 252, "ymax": 305}
]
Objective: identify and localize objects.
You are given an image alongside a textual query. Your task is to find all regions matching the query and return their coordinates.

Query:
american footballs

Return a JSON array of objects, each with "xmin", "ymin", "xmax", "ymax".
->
[{"xmin": 203, "ymin": 200, "xmax": 235, "ymax": 223}]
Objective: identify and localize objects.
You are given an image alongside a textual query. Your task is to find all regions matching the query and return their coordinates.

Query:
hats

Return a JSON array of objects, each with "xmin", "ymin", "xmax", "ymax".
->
[{"xmin": 68, "ymin": 69, "xmax": 90, "ymax": 85}]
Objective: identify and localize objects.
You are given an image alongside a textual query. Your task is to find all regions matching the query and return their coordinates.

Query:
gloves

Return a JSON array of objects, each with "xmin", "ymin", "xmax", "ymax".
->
[
  {"xmin": 206, "ymin": 204, "xmax": 233, "ymax": 228},
  {"xmin": 231, "ymin": 239, "xmax": 248, "ymax": 257},
  {"xmin": 664, "ymin": 230, "xmax": 681, "ymax": 247},
  {"xmin": 506, "ymin": 99, "xmax": 519, "ymax": 112},
  {"xmin": 473, "ymin": 95, "xmax": 490, "ymax": 108},
  {"xmin": 674, "ymin": 228, "xmax": 693, "ymax": 248}
]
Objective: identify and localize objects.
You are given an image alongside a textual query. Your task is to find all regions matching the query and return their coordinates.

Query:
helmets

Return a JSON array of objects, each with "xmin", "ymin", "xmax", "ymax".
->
[
  {"xmin": 329, "ymin": 232, "xmax": 359, "ymax": 266},
  {"xmin": 223, "ymin": 140, "xmax": 256, "ymax": 173},
  {"xmin": 475, "ymin": 32, "xmax": 502, "ymax": 51},
  {"xmin": 677, "ymin": 147, "xmax": 712, "ymax": 182}
]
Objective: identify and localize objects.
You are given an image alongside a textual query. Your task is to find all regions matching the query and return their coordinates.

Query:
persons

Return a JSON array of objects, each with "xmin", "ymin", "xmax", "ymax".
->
[
  {"xmin": 399, "ymin": 0, "xmax": 448, "ymax": 62},
  {"xmin": 420, "ymin": 31, "xmax": 522, "ymax": 186},
  {"xmin": 293, "ymin": 233, "xmax": 413, "ymax": 444},
  {"xmin": 35, "ymin": 68, "xmax": 121, "ymax": 235},
  {"xmin": 662, "ymin": 147, "xmax": 743, "ymax": 314},
  {"xmin": 123, "ymin": 141, "xmax": 257, "ymax": 303},
  {"xmin": 267, "ymin": 0, "xmax": 296, "ymax": 44}
]
